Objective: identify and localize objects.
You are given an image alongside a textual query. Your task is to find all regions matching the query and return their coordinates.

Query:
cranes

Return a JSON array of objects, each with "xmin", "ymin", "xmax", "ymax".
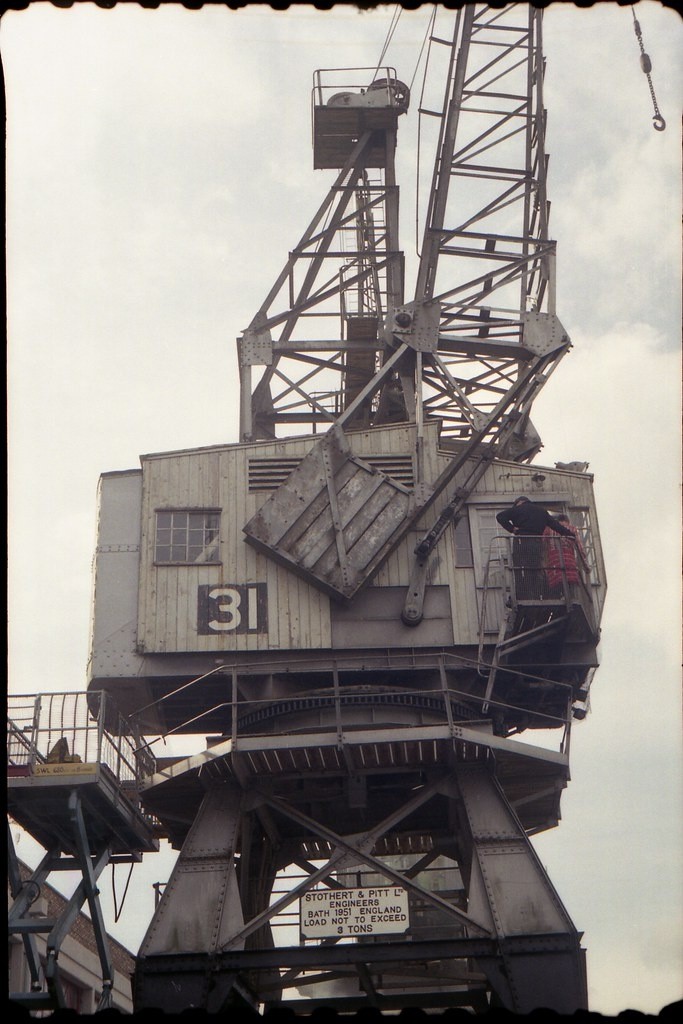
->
[{"xmin": 6, "ymin": 9, "xmax": 664, "ymax": 1009}]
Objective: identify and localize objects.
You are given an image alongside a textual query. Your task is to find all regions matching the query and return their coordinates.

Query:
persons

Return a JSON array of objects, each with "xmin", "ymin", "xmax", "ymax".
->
[
  {"xmin": 496, "ymin": 495, "xmax": 580, "ymax": 605},
  {"xmin": 540, "ymin": 515, "xmax": 591, "ymax": 599}
]
